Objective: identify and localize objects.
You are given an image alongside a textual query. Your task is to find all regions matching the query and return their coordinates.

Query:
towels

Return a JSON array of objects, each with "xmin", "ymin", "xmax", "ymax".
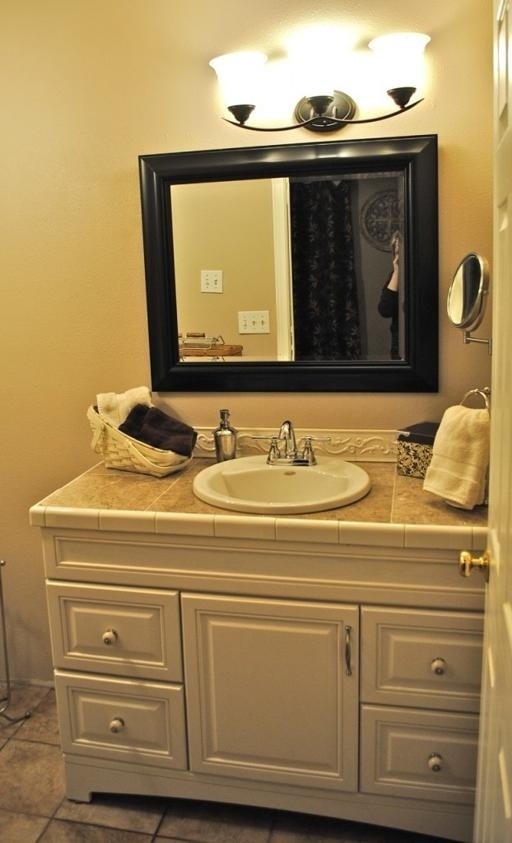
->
[
  {"xmin": 421, "ymin": 404, "xmax": 490, "ymax": 510},
  {"xmin": 95, "ymin": 385, "xmax": 155, "ymax": 428},
  {"xmin": 117, "ymin": 402, "xmax": 198, "ymax": 454}
]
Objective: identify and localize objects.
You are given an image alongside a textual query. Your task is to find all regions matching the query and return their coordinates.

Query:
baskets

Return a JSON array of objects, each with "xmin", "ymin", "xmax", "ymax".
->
[{"xmin": 86, "ymin": 404, "xmax": 194, "ymax": 479}]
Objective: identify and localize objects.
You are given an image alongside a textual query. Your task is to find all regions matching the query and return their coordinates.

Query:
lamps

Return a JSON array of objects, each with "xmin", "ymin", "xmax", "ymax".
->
[{"xmin": 207, "ymin": 23, "xmax": 433, "ymax": 132}]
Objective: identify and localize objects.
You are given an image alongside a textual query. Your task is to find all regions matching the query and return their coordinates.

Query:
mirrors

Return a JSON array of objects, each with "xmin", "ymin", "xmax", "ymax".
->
[
  {"xmin": 135, "ymin": 129, "xmax": 443, "ymax": 397},
  {"xmin": 444, "ymin": 249, "xmax": 492, "ymax": 355}
]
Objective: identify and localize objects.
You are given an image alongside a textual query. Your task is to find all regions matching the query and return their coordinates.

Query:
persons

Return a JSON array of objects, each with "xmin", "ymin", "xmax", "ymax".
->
[{"xmin": 377, "ymin": 231, "xmax": 401, "ymax": 360}]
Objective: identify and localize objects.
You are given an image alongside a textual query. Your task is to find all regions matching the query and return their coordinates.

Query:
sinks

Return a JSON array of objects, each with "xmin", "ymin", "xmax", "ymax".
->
[{"xmin": 192, "ymin": 451, "xmax": 371, "ymax": 514}]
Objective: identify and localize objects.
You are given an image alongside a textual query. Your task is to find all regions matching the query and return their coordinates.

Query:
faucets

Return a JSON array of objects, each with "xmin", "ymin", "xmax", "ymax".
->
[{"xmin": 278, "ymin": 421, "xmax": 297, "ymax": 458}]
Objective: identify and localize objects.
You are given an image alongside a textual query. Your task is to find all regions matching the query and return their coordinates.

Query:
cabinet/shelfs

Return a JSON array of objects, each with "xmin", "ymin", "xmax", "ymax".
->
[
  {"xmin": 42, "ymin": 579, "xmax": 189, "ymax": 840},
  {"xmin": 359, "ymin": 605, "xmax": 484, "ymax": 839},
  {"xmin": 180, "ymin": 589, "xmax": 359, "ymax": 810}
]
